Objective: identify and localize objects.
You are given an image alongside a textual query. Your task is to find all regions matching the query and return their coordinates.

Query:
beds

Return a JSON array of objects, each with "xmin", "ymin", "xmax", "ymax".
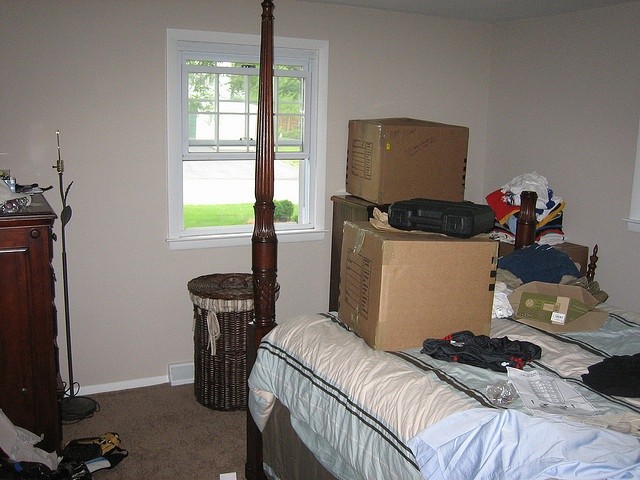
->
[{"xmin": 244, "ymin": 275, "xmax": 640, "ymax": 479}]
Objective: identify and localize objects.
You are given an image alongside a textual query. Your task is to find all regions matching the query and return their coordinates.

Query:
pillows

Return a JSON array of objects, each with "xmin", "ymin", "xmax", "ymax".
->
[{"xmin": 497, "ymin": 242, "xmax": 594, "ymax": 285}]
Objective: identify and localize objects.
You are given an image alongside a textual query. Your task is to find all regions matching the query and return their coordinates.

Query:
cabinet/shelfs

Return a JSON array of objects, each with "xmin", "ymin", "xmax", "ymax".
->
[
  {"xmin": 330, "ymin": 196, "xmax": 390, "ymax": 312},
  {"xmin": 1, "ymin": 184, "xmax": 61, "ymax": 458}
]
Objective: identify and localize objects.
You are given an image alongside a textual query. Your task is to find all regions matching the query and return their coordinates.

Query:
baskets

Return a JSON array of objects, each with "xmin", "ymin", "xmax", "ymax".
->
[{"xmin": 187, "ymin": 272, "xmax": 281, "ymax": 412}]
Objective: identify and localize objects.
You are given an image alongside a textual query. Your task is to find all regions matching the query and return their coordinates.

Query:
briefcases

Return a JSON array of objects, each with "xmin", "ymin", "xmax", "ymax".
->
[{"xmin": 388, "ymin": 196, "xmax": 494, "ymax": 238}]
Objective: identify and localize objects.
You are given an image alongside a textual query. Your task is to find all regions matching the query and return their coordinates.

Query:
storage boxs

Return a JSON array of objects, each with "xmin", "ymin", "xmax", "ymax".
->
[
  {"xmin": 339, "ymin": 221, "xmax": 504, "ymax": 353},
  {"xmin": 345, "ymin": 117, "xmax": 469, "ymax": 206}
]
